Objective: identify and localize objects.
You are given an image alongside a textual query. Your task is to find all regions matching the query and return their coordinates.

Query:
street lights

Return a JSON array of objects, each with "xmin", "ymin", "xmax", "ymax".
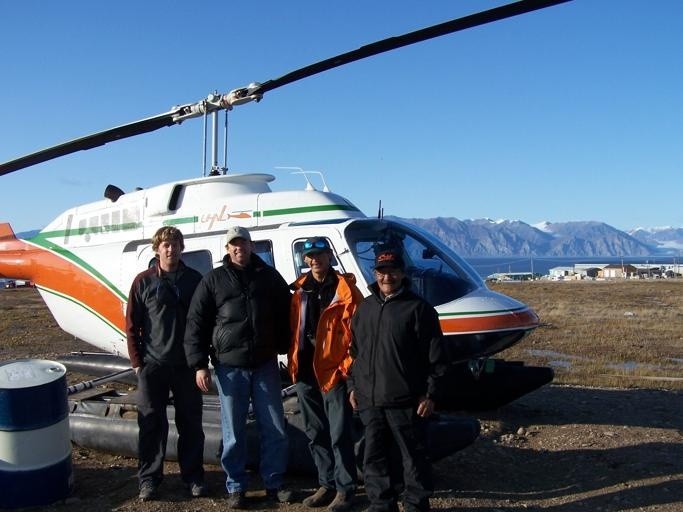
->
[
  {"xmin": 644, "ymin": 260, "xmax": 651, "ymax": 278},
  {"xmin": 620, "ymin": 253, "xmax": 625, "ymax": 274}
]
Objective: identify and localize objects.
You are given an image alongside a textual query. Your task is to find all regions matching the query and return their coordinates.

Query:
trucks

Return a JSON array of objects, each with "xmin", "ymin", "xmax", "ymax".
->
[{"xmin": 5, "ymin": 280, "xmax": 25, "ymax": 288}]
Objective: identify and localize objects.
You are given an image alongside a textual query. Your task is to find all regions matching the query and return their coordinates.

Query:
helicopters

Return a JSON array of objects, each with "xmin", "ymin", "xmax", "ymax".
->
[{"xmin": 0, "ymin": 0, "xmax": 581, "ymax": 470}]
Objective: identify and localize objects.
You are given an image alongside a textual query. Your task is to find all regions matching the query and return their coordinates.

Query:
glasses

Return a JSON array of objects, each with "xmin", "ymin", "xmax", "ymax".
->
[{"xmin": 303, "ymin": 241, "xmax": 328, "ymax": 250}]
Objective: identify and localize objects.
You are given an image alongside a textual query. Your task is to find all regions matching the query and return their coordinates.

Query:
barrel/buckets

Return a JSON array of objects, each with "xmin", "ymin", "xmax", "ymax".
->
[{"xmin": 0, "ymin": 357, "xmax": 73, "ymax": 512}]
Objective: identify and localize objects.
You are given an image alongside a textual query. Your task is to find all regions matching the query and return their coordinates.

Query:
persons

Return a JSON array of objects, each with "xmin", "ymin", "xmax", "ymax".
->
[
  {"xmin": 183, "ymin": 222, "xmax": 294, "ymax": 510},
  {"xmin": 285, "ymin": 235, "xmax": 364, "ymax": 511},
  {"xmin": 344, "ymin": 247, "xmax": 445, "ymax": 512},
  {"xmin": 122, "ymin": 222, "xmax": 208, "ymax": 501}
]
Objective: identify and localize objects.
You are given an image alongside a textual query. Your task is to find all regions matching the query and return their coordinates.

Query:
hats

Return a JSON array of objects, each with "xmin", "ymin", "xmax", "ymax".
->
[
  {"xmin": 302, "ymin": 238, "xmax": 330, "ymax": 257},
  {"xmin": 375, "ymin": 251, "xmax": 404, "ymax": 269},
  {"xmin": 227, "ymin": 227, "xmax": 251, "ymax": 243}
]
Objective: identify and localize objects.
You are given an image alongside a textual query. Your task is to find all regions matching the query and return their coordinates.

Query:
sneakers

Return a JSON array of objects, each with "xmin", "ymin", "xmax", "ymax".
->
[{"xmin": 139, "ymin": 479, "xmax": 355, "ymax": 512}]
